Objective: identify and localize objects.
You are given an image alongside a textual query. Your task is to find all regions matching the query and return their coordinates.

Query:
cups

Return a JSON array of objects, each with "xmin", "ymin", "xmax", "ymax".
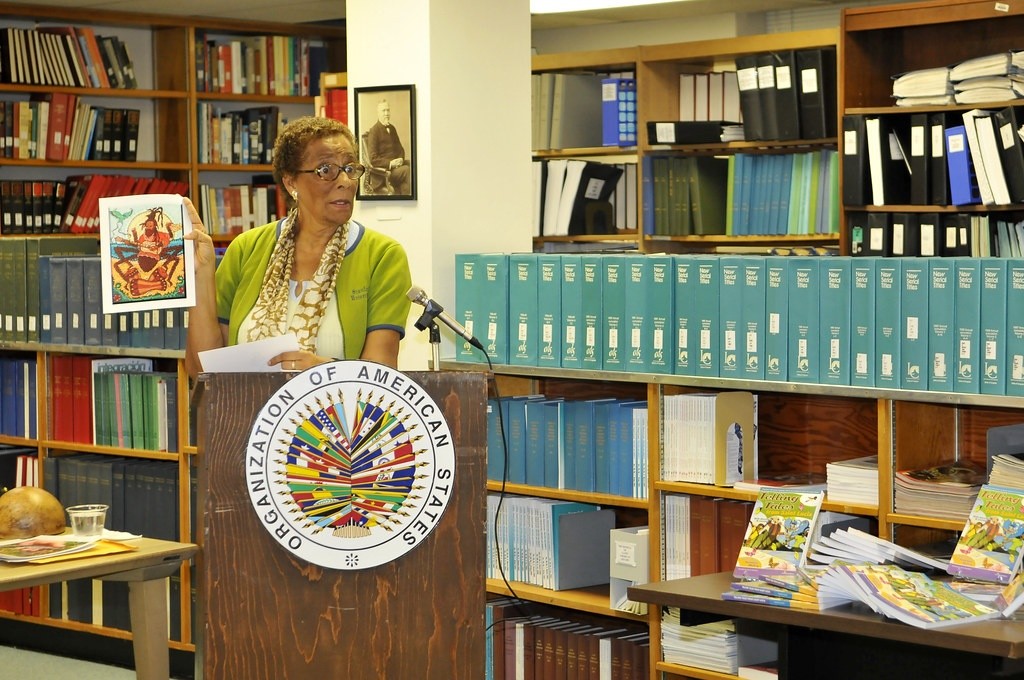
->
[{"xmin": 65, "ymin": 504, "xmax": 110, "ymax": 542}]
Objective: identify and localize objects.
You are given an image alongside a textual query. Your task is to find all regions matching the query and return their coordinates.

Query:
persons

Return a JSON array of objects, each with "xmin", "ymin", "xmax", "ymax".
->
[
  {"xmin": 182, "ymin": 117, "xmax": 413, "ymax": 374},
  {"xmin": 368, "ymin": 99, "xmax": 410, "ymax": 195}
]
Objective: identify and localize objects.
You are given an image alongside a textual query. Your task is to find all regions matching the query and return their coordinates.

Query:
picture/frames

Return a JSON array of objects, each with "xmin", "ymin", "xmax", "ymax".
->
[{"xmin": 354, "ymin": 84, "xmax": 416, "ymax": 200}]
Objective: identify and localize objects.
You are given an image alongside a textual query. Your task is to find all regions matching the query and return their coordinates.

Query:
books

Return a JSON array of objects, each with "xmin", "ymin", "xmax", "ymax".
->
[
  {"xmin": 1, "ymin": 26, "xmax": 189, "ymax": 235},
  {"xmin": 454, "ymin": 51, "xmax": 1024, "ymax": 680},
  {"xmin": 196, "ymin": 31, "xmax": 324, "ymax": 235},
  {"xmin": 2, "ymin": 236, "xmax": 198, "ymax": 650}
]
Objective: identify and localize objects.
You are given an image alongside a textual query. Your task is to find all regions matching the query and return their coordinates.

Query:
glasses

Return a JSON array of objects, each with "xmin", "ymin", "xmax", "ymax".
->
[{"xmin": 295, "ymin": 162, "xmax": 365, "ymax": 181}]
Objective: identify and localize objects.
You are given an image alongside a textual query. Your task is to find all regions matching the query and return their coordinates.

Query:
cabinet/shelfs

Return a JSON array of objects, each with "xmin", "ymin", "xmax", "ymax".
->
[
  {"xmin": 839, "ymin": 0, "xmax": 1023, "ymax": 256},
  {"xmin": 531, "ymin": 29, "xmax": 839, "ymax": 254},
  {"xmin": 0, "ymin": 1, "xmax": 344, "ymax": 651},
  {"xmin": 434, "ymin": 360, "xmax": 1023, "ymax": 679}
]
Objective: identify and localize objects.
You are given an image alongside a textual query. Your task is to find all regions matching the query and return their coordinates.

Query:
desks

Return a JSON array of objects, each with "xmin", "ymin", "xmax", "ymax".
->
[{"xmin": 0, "ymin": 527, "xmax": 201, "ymax": 680}]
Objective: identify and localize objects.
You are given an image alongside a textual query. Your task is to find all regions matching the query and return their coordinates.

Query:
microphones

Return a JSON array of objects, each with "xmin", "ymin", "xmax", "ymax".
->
[{"xmin": 405, "ymin": 285, "xmax": 484, "ymax": 351}]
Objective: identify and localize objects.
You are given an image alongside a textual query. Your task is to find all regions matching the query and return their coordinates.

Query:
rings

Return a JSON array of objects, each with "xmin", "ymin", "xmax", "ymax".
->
[{"xmin": 291, "ymin": 360, "xmax": 295, "ymax": 370}]
